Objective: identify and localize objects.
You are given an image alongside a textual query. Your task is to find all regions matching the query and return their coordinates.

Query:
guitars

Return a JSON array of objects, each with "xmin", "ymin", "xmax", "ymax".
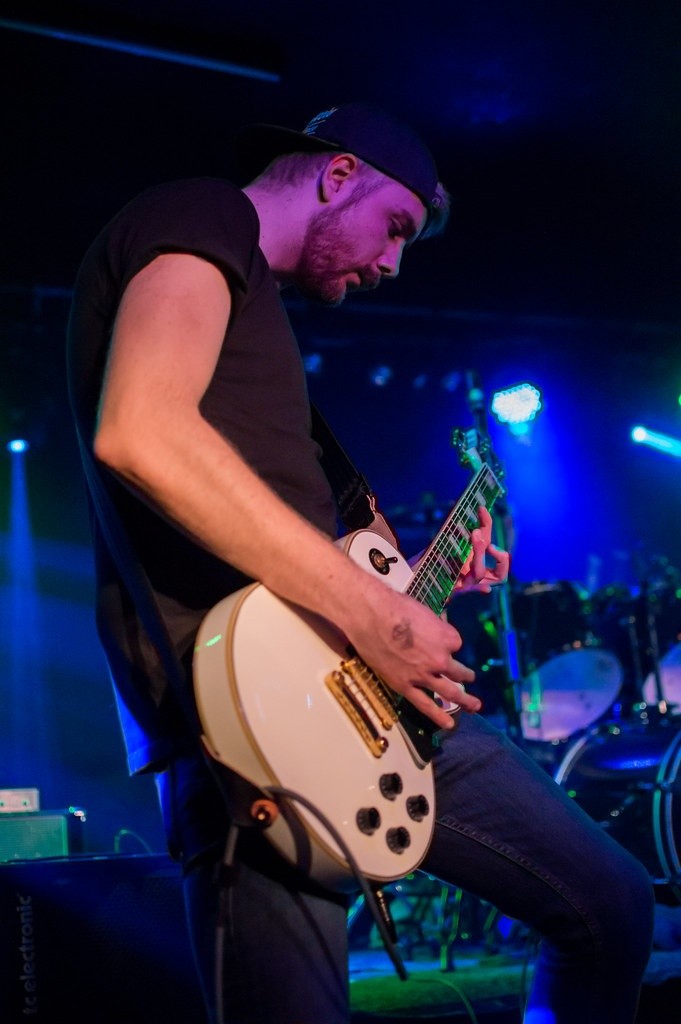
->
[{"xmin": 192, "ymin": 426, "xmax": 505, "ymax": 881}]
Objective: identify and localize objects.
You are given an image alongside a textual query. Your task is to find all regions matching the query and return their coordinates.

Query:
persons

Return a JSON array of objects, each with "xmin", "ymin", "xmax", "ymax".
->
[{"xmin": 65, "ymin": 104, "xmax": 653, "ymax": 1024}]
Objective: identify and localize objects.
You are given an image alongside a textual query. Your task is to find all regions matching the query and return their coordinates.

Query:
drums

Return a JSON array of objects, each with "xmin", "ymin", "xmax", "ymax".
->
[
  {"xmin": 641, "ymin": 642, "xmax": 681, "ymax": 719},
  {"xmin": 554, "ymin": 719, "xmax": 680, "ymax": 905},
  {"xmin": 513, "ymin": 643, "xmax": 624, "ymax": 763}
]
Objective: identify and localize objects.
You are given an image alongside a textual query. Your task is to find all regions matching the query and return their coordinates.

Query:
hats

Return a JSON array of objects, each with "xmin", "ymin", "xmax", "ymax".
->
[{"xmin": 241, "ymin": 103, "xmax": 439, "ymax": 240}]
